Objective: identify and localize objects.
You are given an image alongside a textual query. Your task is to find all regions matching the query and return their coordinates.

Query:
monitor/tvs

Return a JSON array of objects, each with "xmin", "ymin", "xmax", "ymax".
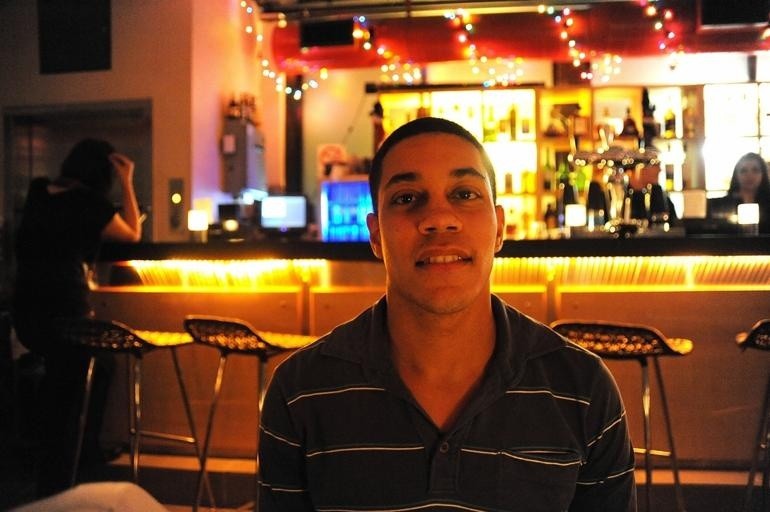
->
[{"xmin": 258, "ymin": 193, "xmax": 309, "ymax": 232}]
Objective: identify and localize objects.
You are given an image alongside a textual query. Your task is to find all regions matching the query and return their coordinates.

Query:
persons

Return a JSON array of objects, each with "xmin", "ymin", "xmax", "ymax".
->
[
  {"xmin": 255, "ymin": 117, "xmax": 637, "ymax": 511},
  {"xmin": 3, "ymin": 134, "xmax": 144, "ymax": 475},
  {"xmin": 543, "ymin": 143, "xmax": 770, "ymax": 238}
]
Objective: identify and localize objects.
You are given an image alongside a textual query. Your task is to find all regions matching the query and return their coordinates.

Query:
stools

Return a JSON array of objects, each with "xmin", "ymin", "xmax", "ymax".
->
[
  {"xmin": 734, "ymin": 319, "xmax": 769, "ymax": 510},
  {"xmin": 183, "ymin": 315, "xmax": 322, "ymax": 512},
  {"xmin": 66, "ymin": 316, "xmax": 215, "ymax": 512},
  {"xmin": 550, "ymin": 318, "xmax": 693, "ymax": 510}
]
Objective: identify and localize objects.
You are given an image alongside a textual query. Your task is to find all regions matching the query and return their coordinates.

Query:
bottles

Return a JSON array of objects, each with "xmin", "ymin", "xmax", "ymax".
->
[{"xmin": 593, "ymin": 107, "xmax": 678, "ymax": 194}]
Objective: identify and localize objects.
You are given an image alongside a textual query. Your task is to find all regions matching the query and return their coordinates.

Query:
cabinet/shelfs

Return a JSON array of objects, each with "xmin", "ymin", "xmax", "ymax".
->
[{"xmin": 533, "ymin": 85, "xmax": 705, "ymax": 231}]
